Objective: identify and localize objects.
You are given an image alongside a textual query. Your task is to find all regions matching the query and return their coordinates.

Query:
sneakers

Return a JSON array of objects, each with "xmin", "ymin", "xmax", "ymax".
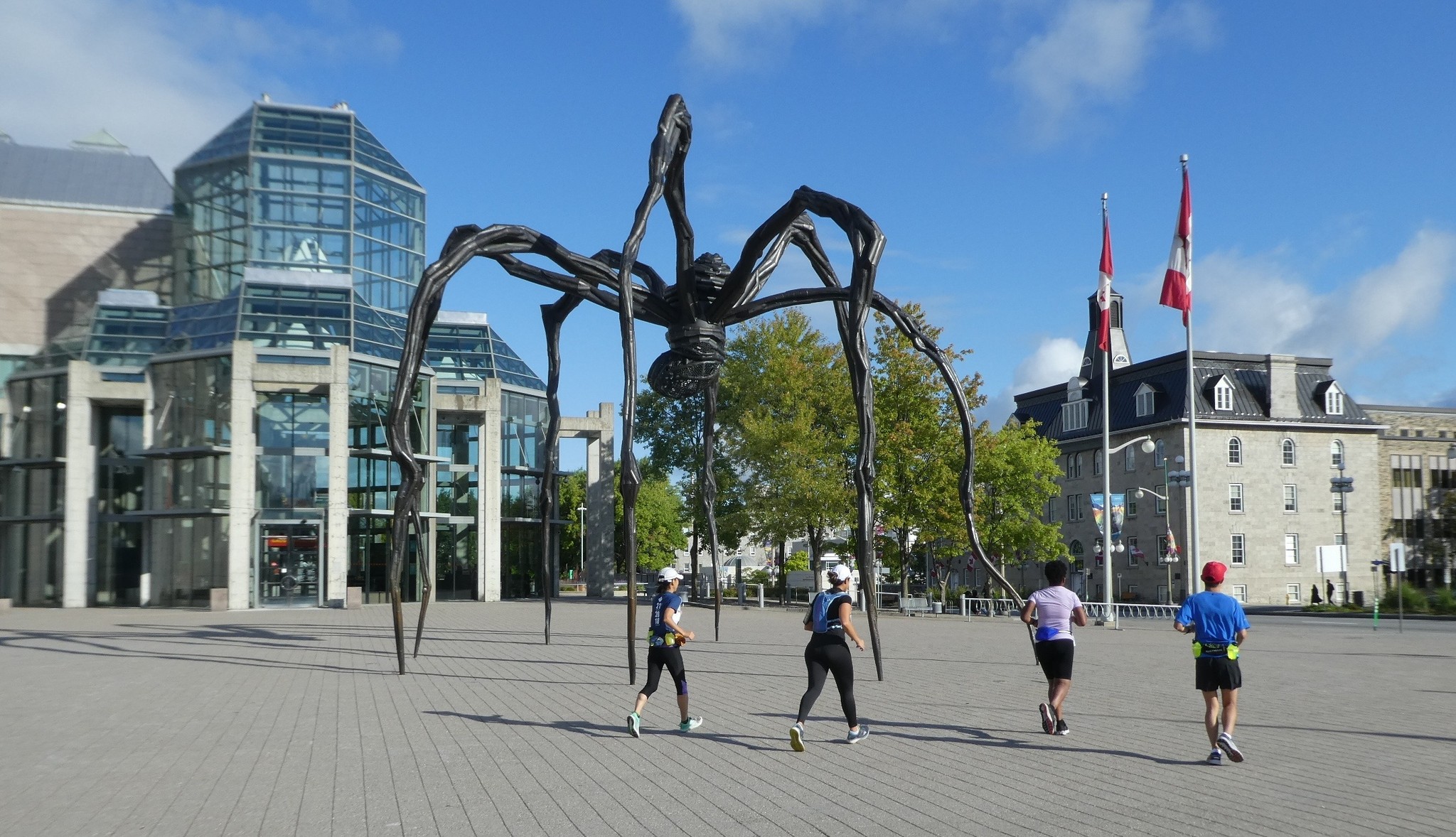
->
[
  {"xmin": 1206, "ymin": 749, "xmax": 1222, "ymax": 765},
  {"xmin": 789, "ymin": 723, "xmax": 805, "ymax": 752},
  {"xmin": 1056, "ymin": 722, "xmax": 1069, "ymax": 736},
  {"xmin": 679, "ymin": 716, "xmax": 703, "ymax": 732},
  {"xmin": 1216, "ymin": 732, "xmax": 1245, "ymax": 763},
  {"xmin": 1039, "ymin": 703, "xmax": 1057, "ymax": 735},
  {"xmin": 846, "ymin": 725, "xmax": 870, "ymax": 744},
  {"xmin": 627, "ymin": 712, "xmax": 641, "ymax": 738}
]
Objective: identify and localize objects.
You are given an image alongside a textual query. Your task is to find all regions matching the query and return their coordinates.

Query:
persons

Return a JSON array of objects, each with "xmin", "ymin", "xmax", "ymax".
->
[
  {"xmin": 627, "ymin": 568, "xmax": 703, "ymax": 737},
  {"xmin": 1020, "ymin": 561, "xmax": 1086, "ymax": 734},
  {"xmin": 1311, "ymin": 585, "xmax": 1322, "ymax": 605},
  {"xmin": 1327, "ymin": 579, "xmax": 1335, "ymax": 605},
  {"xmin": 1173, "ymin": 562, "xmax": 1250, "ymax": 766},
  {"xmin": 789, "ymin": 564, "xmax": 870, "ymax": 752}
]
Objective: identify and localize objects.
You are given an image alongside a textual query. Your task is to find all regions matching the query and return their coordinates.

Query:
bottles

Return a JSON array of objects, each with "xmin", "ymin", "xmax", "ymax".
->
[
  {"xmin": 665, "ymin": 630, "xmax": 675, "ymax": 645},
  {"xmin": 1228, "ymin": 641, "xmax": 1239, "ymax": 660},
  {"xmin": 646, "ymin": 627, "xmax": 653, "ymax": 643},
  {"xmin": 1192, "ymin": 638, "xmax": 1202, "ymax": 659}
]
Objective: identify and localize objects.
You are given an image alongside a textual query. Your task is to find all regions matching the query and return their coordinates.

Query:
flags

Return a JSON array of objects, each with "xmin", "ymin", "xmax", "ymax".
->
[
  {"xmin": 1159, "ymin": 169, "xmax": 1192, "ymax": 328},
  {"xmin": 1097, "ymin": 215, "xmax": 1114, "ymax": 351},
  {"xmin": 1130, "ymin": 546, "xmax": 1144, "ymax": 560}
]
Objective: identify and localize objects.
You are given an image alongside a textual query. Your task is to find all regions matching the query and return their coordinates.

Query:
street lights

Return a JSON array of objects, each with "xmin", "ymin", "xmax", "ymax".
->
[
  {"xmin": 1094, "ymin": 434, "xmax": 1156, "ymax": 627},
  {"xmin": 1167, "ymin": 454, "xmax": 1191, "ymax": 606},
  {"xmin": 1329, "ymin": 463, "xmax": 1354, "ymax": 604},
  {"xmin": 576, "ymin": 502, "xmax": 587, "ymax": 570},
  {"xmin": 1135, "ymin": 458, "xmax": 1174, "ymax": 605}
]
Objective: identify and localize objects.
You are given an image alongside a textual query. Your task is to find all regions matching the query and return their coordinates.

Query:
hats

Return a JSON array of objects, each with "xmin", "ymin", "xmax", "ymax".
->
[
  {"xmin": 657, "ymin": 567, "xmax": 684, "ymax": 583},
  {"xmin": 1200, "ymin": 562, "xmax": 1227, "ymax": 584},
  {"xmin": 831, "ymin": 565, "xmax": 852, "ymax": 581}
]
